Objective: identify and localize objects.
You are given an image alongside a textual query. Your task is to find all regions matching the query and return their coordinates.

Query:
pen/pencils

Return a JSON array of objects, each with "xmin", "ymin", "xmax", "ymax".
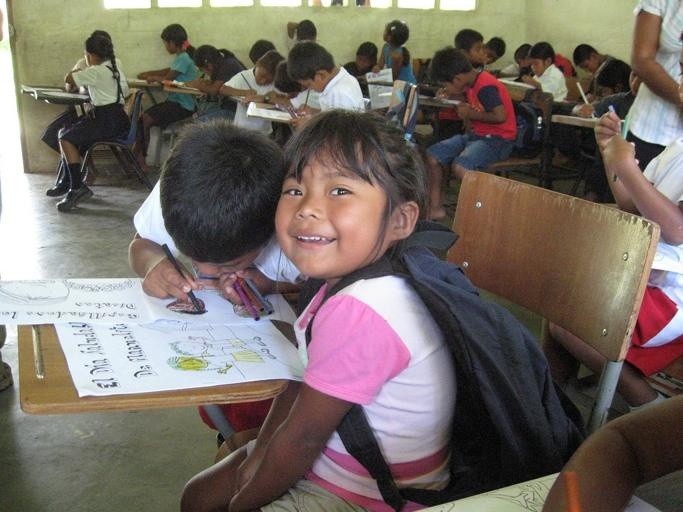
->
[
  {"xmin": 609, "ymin": 105, "xmax": 622, "ymax": 134},
  {"xmin": 576, "ymin": 82, "xmax": 595, "ymax": 119},
  {"xmin": 613, "ymin": 113, "xmax": 631, "ymax": 182},
  {"xmin": 162, "ymin": 243, "xmax": 200, "ymax": 311},
  {"xmin": 303, "ymin": 88, "xmax": 310, "ymax": 111},
  {"xmin": 241, "ymin": 73, "xmax": 251, "ymax": 89},
  {"xmin": 233, "ymin": 272, "xmax": 272, "ymax": 321},
  {"xmin": 286, "ymin": 105, "xmax": 298, "ymax": 120}
]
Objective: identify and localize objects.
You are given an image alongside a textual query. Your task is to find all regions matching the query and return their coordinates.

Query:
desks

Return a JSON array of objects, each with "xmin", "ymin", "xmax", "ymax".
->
[
  {"xmin": 411, "ymin": 471, "xmax": 663, "ymax": 512},
  {"xmin": 18, "ymin": 279, "xmax": 290, "ymax": 451}
]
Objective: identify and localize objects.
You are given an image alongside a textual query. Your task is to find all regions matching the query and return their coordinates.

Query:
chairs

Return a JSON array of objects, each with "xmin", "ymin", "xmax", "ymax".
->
[
  {"xmin": 21, "ymin": 58, "xmax": 628, "ymax": 223},
  {"xmin": 212, "ymin": 170, "xmax": 662, "ymax": 463}
]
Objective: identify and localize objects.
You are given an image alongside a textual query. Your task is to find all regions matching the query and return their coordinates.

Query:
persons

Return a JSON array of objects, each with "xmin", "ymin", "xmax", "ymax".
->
[
  {"xmin": 538, "ymin": 104, "xmax": 682, "ymax": 414},
  {"xmin": 176, "ymin": 105, "xmax": 460, "ymax": 512},
  {"xmin": 622, "ymin": 1, "xmax": 683, "ymax": 176},
  {"xmin": 40, "ymin": 30, "xmax": 129, "ymax": 174},
  {"xmin": 42, "ymin": 30, "xmax": 129, "ymax": 214},
  {"xmin": 539, "ymin": 390, "xmax": 683, "ymax": 511},
  {"xmin": 126, "ymin": 119, "xmax": 308, "ymax": 445},
  {"xmin": 130, "ymin": 15, "xmax": 645, "ymax": 222}
]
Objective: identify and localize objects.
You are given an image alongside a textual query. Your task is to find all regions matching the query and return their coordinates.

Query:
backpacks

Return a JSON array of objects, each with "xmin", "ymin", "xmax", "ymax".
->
[
  {"xmin": 513, "ymin": 86, "xmax": 544, "ymax": 159},
  {"xmin": 309, "ymin": 219, "xmax": 584, "ymax": 511},
  {"xmin": 386, "ymin": 81, "xmax": 419, "ymax": 141}
]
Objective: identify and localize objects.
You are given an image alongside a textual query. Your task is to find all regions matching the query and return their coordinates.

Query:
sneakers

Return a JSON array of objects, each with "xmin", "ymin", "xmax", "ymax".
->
[
  {"xmin": 46, "ymin": 180, "xmax": 69, "ymax": 197},
  {"xmin": 57, "ymin": 185, "xmax": 93, "ymax": 212}
]
